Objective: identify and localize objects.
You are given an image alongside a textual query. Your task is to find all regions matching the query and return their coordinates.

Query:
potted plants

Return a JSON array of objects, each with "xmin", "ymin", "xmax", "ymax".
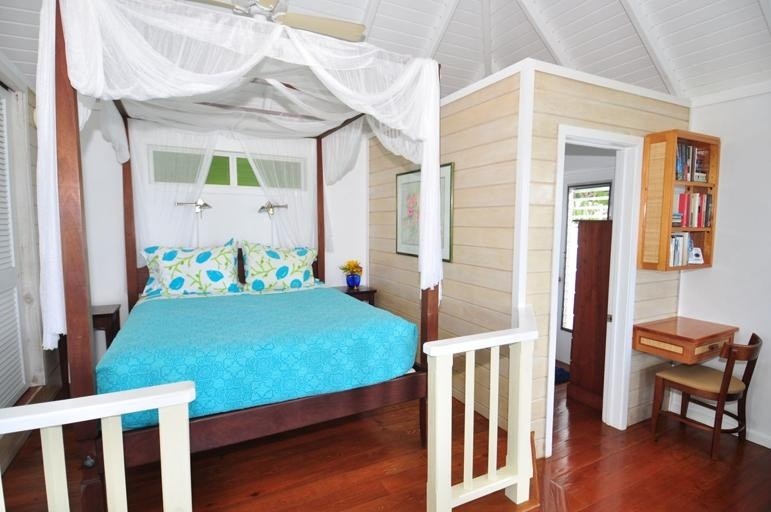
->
[{"xmin": 396, "ymin": 160, "xmax": 454, "ymax": 264}]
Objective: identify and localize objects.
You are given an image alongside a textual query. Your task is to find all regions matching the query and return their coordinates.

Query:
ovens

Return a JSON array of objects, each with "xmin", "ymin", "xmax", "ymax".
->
[{"xmin": 80, "ymin": 266, "xmax": 440, "ymax": 506}]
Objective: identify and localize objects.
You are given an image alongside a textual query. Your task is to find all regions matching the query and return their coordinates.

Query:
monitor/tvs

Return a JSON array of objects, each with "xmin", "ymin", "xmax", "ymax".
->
[{"xmin": 669, "ymin": 140, "xmax": 715, "ymax": 268}]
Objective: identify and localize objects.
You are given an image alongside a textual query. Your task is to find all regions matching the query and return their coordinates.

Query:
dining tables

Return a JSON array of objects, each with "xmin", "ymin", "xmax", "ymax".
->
[{"xmin": 339, "ymin": 259, "xmax": 365, "ymax": 289}]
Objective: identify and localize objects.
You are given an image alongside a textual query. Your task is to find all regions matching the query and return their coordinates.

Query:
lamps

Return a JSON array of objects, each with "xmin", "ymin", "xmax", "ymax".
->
[{"xmin": 647, "ymin": 332, "xmax": 764, "ymax": 460}]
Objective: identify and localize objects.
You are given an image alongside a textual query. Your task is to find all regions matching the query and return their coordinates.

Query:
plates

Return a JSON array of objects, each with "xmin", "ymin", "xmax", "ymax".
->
[{"xmin": 638, "ymin": 127, "xmax": 720, "ymax": 272}]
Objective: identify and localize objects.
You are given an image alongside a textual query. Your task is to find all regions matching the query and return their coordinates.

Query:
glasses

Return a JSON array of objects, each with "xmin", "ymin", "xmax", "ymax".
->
[
  {"xmin": 258, "ymin": 199, "xmax": 288, "ymax": 217},
  {"xmin": 177, "ymin": 198, "xmax": 212, "ymax": 215}
]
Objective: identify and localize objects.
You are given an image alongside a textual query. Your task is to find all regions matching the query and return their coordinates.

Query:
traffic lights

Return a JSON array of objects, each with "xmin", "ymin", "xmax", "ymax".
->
[{"xmin": 140, "ymin": 243, "xmax": 320, "ymax": 297}]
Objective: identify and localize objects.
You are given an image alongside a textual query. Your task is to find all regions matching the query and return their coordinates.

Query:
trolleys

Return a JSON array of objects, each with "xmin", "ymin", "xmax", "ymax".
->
[{"xmin": 183, "ymin": 0, "xmax": 369, "ymax": 44}]
